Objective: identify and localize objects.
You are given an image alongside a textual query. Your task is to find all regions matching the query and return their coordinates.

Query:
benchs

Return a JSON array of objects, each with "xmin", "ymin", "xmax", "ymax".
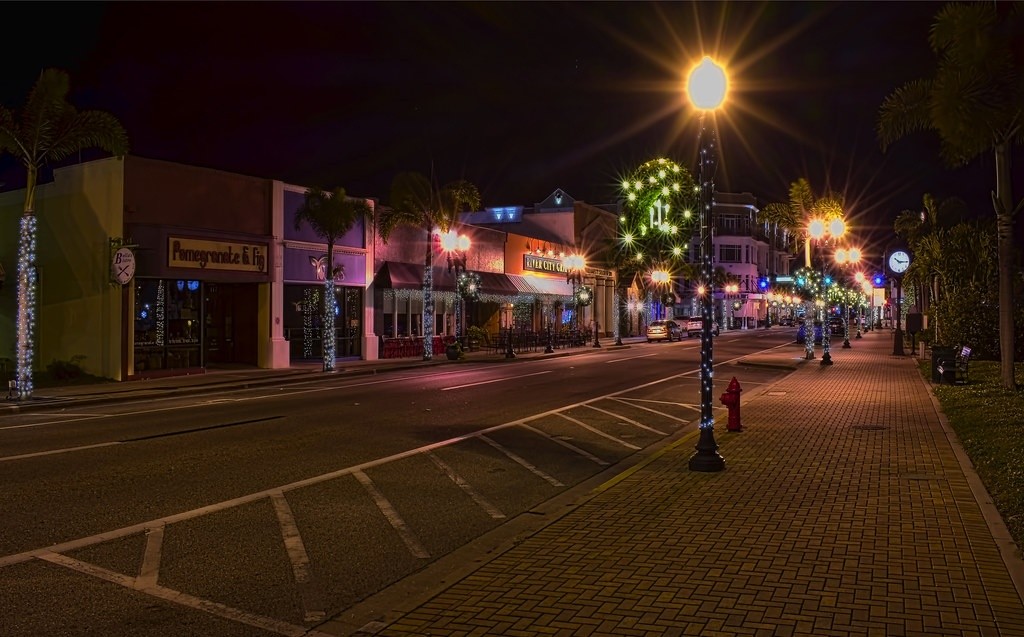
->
[{"xmin": 936, "ymin": 346, "xmax": 971, "ymax": 386}]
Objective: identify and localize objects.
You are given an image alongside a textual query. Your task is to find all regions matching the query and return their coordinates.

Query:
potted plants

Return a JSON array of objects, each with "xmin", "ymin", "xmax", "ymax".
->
[
  {"xmin": 443, "ymin": 339, "xmax": 467, "ymax": 362},
  {"xmin": 464, "ymin": 325, "xmax": 490, "ymax": 353},
  {"xmin": 46, "ymin": 353, "xmax": 87, "ymax": 384}
]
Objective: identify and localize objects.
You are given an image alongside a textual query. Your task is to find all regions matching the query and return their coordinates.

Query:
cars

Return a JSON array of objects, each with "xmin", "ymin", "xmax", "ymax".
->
[
  {"xmin": 798, "ymin": 320, "xmax": 832, "ymax": 344},
  {"xmin": 646, "ymin": 320, "xmax": 682, "ymax": 343},
  {"xmin": 686, "ymin": 316, "xmax": 720, "ymax": 338},
  {"xmin": 828, "ymin": 317, "xmax": 846, "ymax": 337},
  {"xmin": 674, "ymin": 315, "xmax": 690, "ymax": 331},
  {"xmin": 853, "ymin": 314, "xmax": 865, "ymax": 326}
]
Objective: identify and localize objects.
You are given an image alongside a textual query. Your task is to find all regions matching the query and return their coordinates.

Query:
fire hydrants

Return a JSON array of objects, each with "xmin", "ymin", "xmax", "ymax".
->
[{"xmin": 718, "ymin": 377, "xmax": 742, "ymax": 432}]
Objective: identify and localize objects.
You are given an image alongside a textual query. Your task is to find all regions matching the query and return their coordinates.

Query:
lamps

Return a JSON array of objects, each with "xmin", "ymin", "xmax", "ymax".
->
[{"xmin": 529, "ymin": 237, "xmax": 573, "ymax": 259}]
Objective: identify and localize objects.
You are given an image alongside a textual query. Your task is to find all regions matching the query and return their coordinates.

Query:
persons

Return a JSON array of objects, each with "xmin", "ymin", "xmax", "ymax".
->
[{"xmin": 589, "ymin": 320, "xmax": 595, "ymax": 339}]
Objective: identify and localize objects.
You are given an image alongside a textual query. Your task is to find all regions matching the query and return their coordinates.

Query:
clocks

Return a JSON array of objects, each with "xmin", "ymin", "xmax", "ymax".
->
[{"xmin": 888, "ymin": 248, "xmax": 912, "ymax": 276}]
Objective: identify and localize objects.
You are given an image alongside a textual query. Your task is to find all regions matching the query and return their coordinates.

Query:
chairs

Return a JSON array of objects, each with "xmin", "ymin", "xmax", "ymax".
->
[{"xmin": 482, "ymin": 329, "xmax": 588, "ymax": 356}]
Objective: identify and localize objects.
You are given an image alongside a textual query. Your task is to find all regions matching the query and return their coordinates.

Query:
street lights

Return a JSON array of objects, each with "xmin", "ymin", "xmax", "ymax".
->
[
  {"xmin": 563, "ymin": 254, "xmax": 585, "ymax": 328},
  {"xmin": 687, "ymin": 55, "xmax": 726, "ymax": 472},
  {"xmin": 765, "ymin": 293, "xmax": 772, "ymax": 327},
  {"xmin": 811, "ymin": 220, "xmax": 846, "ymax": 365},
  {"xmin": 855, "ymin": 272, "xmax": 864, "ymax": 339},
  {"xmin": 441, "ymin": 232, "xmax": 471, "ymax": 352},
  {"xmin": 725, "ymin": 285, "xmax": 738, "ymax": 329},
  {"xmin": 834, "ymin": 248, "xmax": 861, "ymax": 348}
]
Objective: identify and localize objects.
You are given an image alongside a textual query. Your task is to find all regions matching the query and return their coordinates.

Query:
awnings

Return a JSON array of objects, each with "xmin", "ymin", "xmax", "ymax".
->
[{"xmin": 385, "ymin": 261, "xmax": 573, "ymax": 296}]
